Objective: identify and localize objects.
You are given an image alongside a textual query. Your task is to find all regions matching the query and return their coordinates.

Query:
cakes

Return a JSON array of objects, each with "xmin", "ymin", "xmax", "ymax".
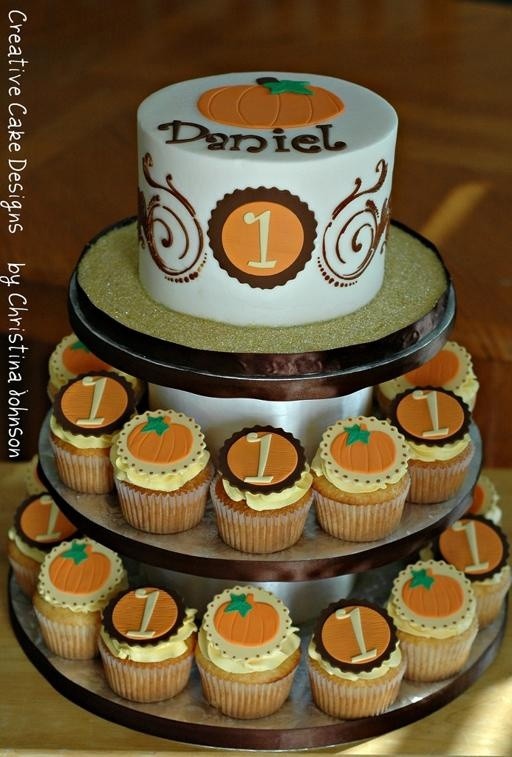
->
[{"xmin": 5, "ymin": 70, "xmax": 512, "ymax": 750}]
